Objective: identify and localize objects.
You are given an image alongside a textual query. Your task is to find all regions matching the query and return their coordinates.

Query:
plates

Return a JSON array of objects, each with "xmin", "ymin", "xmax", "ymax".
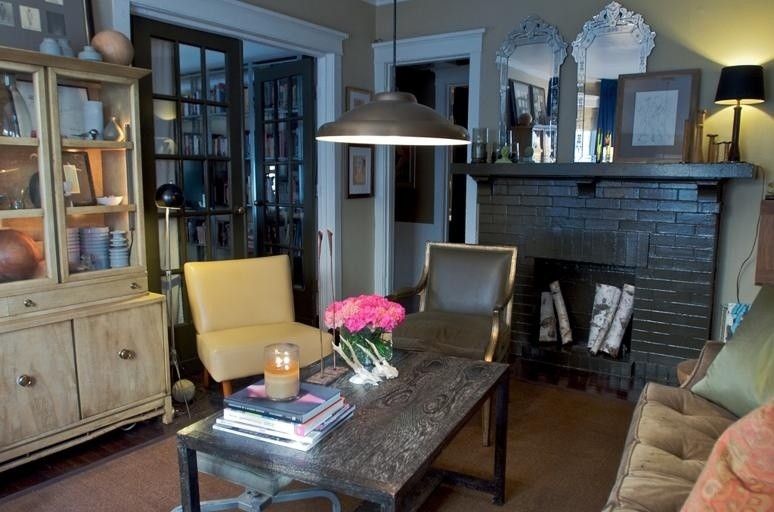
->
[{"xmin": 80, "ymin": 225, "xmax": 130, "ymax": 268}]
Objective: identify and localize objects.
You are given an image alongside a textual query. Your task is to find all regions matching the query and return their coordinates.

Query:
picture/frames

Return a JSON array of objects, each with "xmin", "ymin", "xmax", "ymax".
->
[
  {"xmin": 0, "ymin": 0, "xmax": 93, "ymax": 58},
  {"xmin": 347, "ymin": 85, "xmax": 373, "ymax": 113},
  {"xmin": 347, "ymin": 144, "xmax": 377, "ymax": 200},
  {"xmin": 508, "ymin": 78, "xmax": 530, "ymax": 126},
  {"xmin": 612, "ymin": 68, "xmax": 700, "ymax": 164},
  {"xmin": 530, "ymin": 84, "xmax": 548, "ymax": 123}
]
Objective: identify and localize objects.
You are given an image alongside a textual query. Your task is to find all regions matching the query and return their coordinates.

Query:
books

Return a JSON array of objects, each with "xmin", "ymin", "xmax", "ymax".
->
[
  {"xmin": 177, "ymin": 76, "xmax": 302, "ymax": 288},
  {"xmin": 719, "ymin": 301, "xmax": 753, "ymax": 345},
  {"xmin": 211, "ymin": 374, "xmax": 356, "ymax": 451}
]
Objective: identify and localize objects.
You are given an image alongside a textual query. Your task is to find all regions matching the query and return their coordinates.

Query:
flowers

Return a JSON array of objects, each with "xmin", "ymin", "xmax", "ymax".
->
[{"xmin": 322, "ymin": 293, "xmax": 406, "ymax": 360}]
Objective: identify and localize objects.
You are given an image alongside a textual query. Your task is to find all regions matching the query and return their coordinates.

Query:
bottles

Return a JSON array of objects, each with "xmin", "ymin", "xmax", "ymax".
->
[
  {"xmin": 102, "ymin": 116, "xmax": 123, "ymax": 141},
  {"xmin": 39, "ymin": 38, "xmax": 102, "ymax": 61}
]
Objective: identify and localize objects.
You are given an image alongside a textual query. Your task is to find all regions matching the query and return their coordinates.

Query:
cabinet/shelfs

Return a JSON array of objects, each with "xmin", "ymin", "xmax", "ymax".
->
[{"xmin": 178, "ymin": 57, "xmax": 308, "ymax": 290}]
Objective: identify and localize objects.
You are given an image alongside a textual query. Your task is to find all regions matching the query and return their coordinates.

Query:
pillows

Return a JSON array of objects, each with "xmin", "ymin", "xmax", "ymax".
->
[
  {"xmin": 690, "ymin": 284, "xmax": 773, "ymax": 417},
  {"xmin": 677, "ymin": 399, "xmax": 773, "ymax": 511}
]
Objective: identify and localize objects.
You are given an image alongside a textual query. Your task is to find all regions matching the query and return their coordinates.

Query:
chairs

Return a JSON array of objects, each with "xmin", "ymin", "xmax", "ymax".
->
[
  {"xmin": 386, "ymin": 241, "xmax": 518, "ymax": 447},
  {"xmin": 178, "ymin": 252, "xmax": 335, "ymax": 405}
]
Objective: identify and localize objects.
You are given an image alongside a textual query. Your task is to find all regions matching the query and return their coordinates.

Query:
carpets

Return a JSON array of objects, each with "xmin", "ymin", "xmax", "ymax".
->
[{"xmin": 1, "ymin": 380, "xmax": 635, "ymax": 512}]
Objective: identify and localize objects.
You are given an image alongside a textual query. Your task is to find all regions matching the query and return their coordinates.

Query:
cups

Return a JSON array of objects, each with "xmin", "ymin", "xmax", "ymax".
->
[
  {"xmin": 471, "ymin": 128, "xmax": 488, "ymax": 163},
  {"xmin": 262, "ymin": 342, "xmax": 300, "ymax": 403},
  {"xmin": 5, "ymin": 184, "xmax": 27, "ymax": 208}
]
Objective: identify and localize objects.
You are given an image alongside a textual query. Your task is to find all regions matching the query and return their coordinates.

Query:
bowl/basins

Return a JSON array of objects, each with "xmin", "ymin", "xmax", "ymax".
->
[
  {"xmin": 66, "ymin": 227, "xmax": 80, "ymax": 267},
  {"xmin": 96, "ymin": 196, "xmax": 123, "ymax": 207}
]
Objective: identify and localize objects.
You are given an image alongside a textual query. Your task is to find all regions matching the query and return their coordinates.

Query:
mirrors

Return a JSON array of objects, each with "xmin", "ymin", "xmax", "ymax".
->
[
  {"xmin": 568, "ymin": 1, "xmax": 657, "ymax": 164},
  {"xmin": 495, "ymin": 14, "xmax": 568, "ymax": 164}
]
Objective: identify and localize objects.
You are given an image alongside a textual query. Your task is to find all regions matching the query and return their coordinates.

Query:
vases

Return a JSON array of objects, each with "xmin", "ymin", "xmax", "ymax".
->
[{"xmin": 341, "ymin": 326, "xmax": 394, "ymax": 364}]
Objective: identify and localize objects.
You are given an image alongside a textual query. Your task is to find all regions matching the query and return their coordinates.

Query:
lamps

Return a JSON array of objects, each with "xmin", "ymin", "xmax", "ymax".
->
[
  {"xmin": 316, "ymin": 0, "xmax": 471, "ymax": 146},
  {"xmin": 714, "ymin": 63, "xmax": 766, "ymax": 161}
]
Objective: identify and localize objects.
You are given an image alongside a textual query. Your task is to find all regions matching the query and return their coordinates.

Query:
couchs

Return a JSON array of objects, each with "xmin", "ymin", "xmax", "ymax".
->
[{"xmin": 594, "ymin": 337, "xmax": 774, "ymax": 512}]
